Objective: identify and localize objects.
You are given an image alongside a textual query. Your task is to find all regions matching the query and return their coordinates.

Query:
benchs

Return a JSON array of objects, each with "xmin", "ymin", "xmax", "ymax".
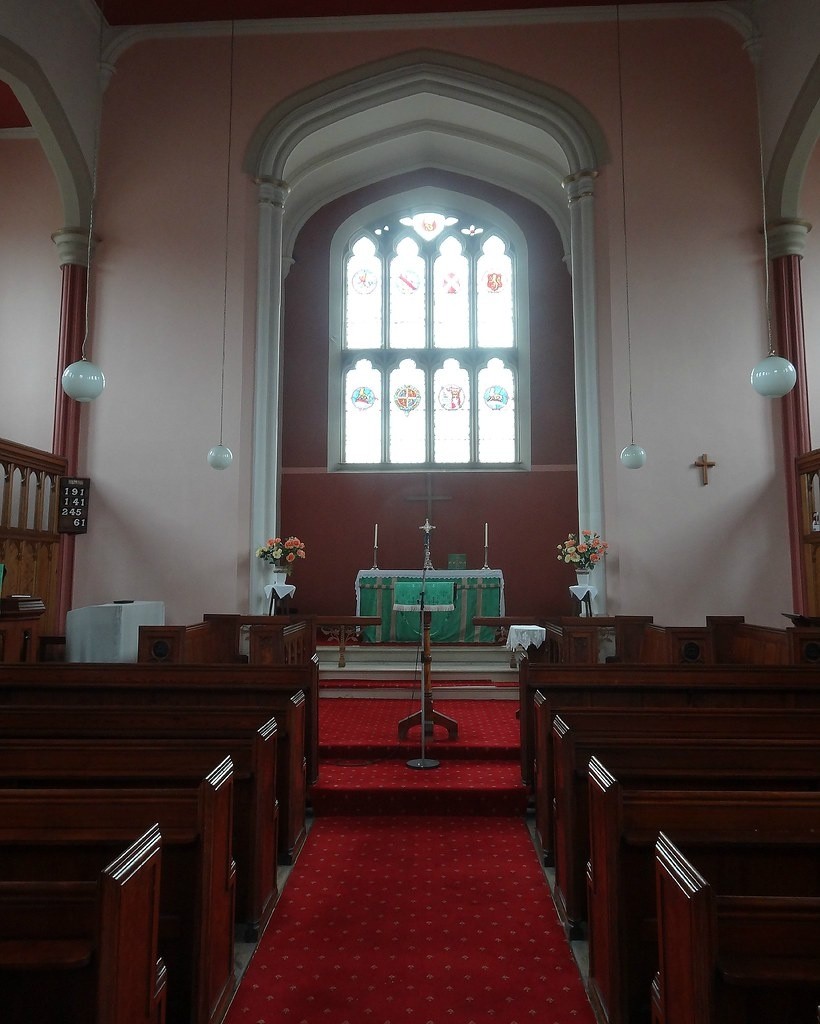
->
[
  {"xmin": 241, "ymin": 616, "xmax": 380, "ymax": 667},
  {"xmin": 517, "ymin": 659, "xmax": 819, "ymax": 1024},
  {"xmin": 471, "ymin": 615, "xmax": 655, "ymax": 668},
  {"xmin": 0, "ymin": 661, "xmax": 321, "ymax": 1024}
]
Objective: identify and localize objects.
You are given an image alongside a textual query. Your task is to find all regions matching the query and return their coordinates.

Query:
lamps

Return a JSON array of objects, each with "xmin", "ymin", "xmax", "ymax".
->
[
  {"xmin": 750, "ymin": 50, "xmax": 797, "ymax": 399},
  {"xmin": 62, "ymin": 6, "xmax": 105, "ymax": 403},
  {"xmin": 207, "ymin": 18, "xmax": 236, "ymax": 470},
  {"xmin": 616, "ymin": 0, "xmax": 645, "ymax": 470}
]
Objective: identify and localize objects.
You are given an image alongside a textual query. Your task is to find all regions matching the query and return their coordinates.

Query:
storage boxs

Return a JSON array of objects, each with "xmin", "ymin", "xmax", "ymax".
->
[{"xmin": 447, "ymin": 553, "xmax": 467, "ymax": 570}]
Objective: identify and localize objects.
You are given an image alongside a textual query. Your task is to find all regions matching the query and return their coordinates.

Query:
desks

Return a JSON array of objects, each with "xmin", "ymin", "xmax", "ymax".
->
[
  {"xmin": 354, "ymin": 570, "xmax": 505, "ymax": 644},
  {"xmin": 505, "ymin": 624, "xmax": 548, "ymax": 667},
  {"xmin": 264, "ymin": 585, "xmax": 296, "ymax": 615},
  {"xmin": 568, "ymin": 585, "xmax": 597, "ymax": 617},
  {"xmin": 65, "ymin": 601, "xmax": 166, "ymax": 665}
]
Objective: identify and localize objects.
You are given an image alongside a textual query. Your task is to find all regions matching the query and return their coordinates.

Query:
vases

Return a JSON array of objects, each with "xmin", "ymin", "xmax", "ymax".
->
[
  {"xmin": 575, "ymin": 571, "xmax": 590, "ymax": 586},
  {"xmin": 274, "ymin": 569, "xmax": 287, "ymax": 585}
]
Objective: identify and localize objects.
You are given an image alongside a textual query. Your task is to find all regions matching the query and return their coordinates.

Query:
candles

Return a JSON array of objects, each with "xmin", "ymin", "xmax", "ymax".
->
[
  {"xmin": 484, "ymin": 523, "xmax": 489, "ymax": 547},
  {"xmin": 374, "ymin": 524, "xmax": 378, "ymax": 548}
]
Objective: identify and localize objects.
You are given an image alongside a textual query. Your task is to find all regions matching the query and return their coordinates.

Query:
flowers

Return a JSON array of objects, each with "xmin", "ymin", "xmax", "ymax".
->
[
  {"xmin": 255, "ymin": 536, "xmax": 306, "ymax": 575},
  {"xmin": 557, "ymin": 530, "xmax": 608, "ymax": 575}
]
